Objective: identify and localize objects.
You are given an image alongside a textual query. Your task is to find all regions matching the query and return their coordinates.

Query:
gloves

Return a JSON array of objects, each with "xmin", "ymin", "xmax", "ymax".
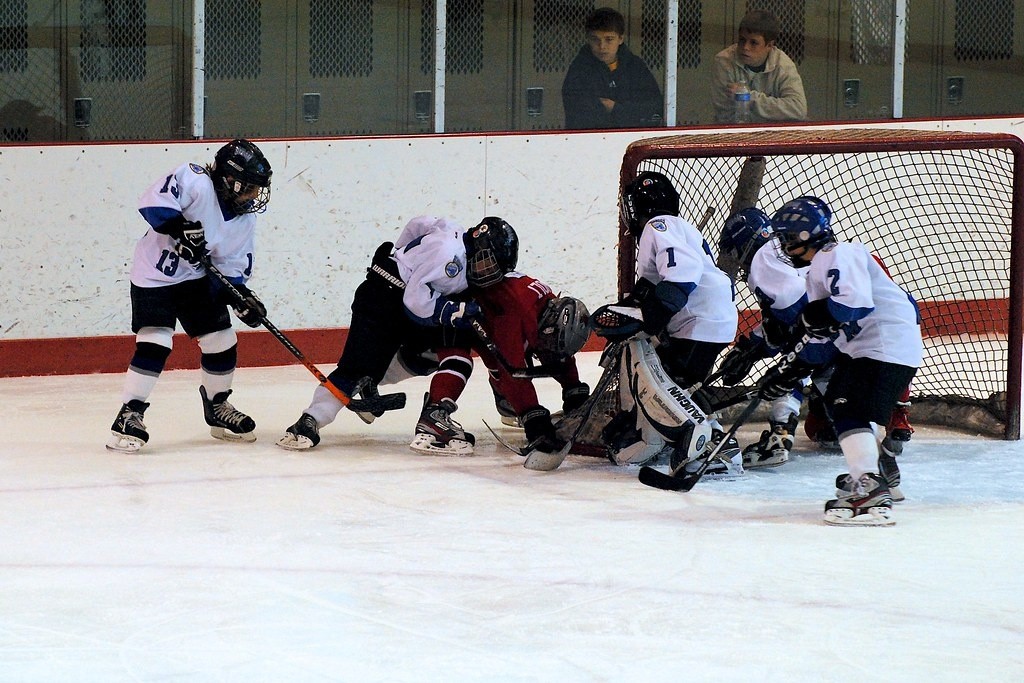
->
[
  {"xmin": 757, "ymin": 351, "xmax": 814, "ymax": 402},
  {"xmin": 370, "ymin": 242, "xmax": 396, "ymax": 268},
  {"xmin": 227, "ymin": 283, "xmax": 267, "ymax": 328},
  {"xmin": 174, "ymin": 221, "xmax": 211, "ymax": 270},
  {"xmin": 561, "ymin": 381, "xmax": 591, "ymax": 413},
  {"xmin": 518, "ymin": 404, "xmax": 565, "ymax": 454},
  {"xmin": 718, "ymin": 333, "xmax": 761, "ymax": 387},
  {"xmin": 799, "ymin": 302, "xmax": 837, "ymax": 339},
  {"xmin": 434, "ymin": 299, "xmax": 484, "ymax": 328}
]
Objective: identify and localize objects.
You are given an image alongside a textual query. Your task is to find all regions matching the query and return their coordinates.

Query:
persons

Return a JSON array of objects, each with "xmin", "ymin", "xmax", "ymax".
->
[
  {"xmin": 562, "ymin": 7, "xmax": 663, "ymax": 129},
  {"xmin": 589, "ymin": 170, "xmax": 743, "ymax": 471},
  {"xmin": 286, "ymin": 216, "xmax": 519, "ymax": 446},
  {"xmin": 712, "ymin": 10, "xmax": 807, "ymax": 124},
  {"xmin": 718, "ymin": 205, "xmax": 923, "ymax": 518},
  {"xmin": 110, "ymin": 139, "xmax": 273, "ymax": 446},
  {"xmin": 377, "ymin": 272, "xmax": 591, "ymax": 470}
]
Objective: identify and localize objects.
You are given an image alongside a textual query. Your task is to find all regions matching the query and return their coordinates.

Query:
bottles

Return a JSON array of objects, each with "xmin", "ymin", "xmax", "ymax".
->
[{"xmin": 734, "ymin": 76, "xmax": 751, "ymax": 125}]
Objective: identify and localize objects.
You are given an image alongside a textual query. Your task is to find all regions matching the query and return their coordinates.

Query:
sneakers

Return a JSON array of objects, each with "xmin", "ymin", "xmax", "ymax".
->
[
  {"xmin": 409, "ymin": 392, "xmax": 476, "ymax": 454},
  {"xmin": 199, "ymin": 384, "xmax": 257, "ymax": 443},
  {"xmin": 106, "ymin": 399, "xmax": 150, "ymax": 453},
  {"xmin": 742, "ymin": 412, "xmax": 798, "ymax": 469},
  {"xmin": 276, "ymin": 413, "xmax": 320, "ymax": 451},
  {"xmin": 881, "ymin": 434, "xmax": 904, "ymax": 457},
  {"xmin": 835, "ymin": 437, "xmax": 905, "ymax": 502},
  {"xmin": 684, "ymin": 429, "xmax": 745, "ymax": 480},
  {"xmin": 890, "ymin": 426, "xmax": 911, "ymax": 440},
  {"xmin": 823, "ymin": 471, "xmax": 896, "ymax": 527}
]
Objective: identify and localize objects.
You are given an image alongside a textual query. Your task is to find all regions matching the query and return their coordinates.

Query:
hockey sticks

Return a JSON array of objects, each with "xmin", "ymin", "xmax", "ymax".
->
[
  {"xmin": 483, "ymin": 421, "xmax": 542, "ymax": 457},
  {"xmin": 639, "ymin": 393, "xmax": 764, "ymax": 492},
  {"xmin": 700, "ymin": 338, "xmax": 766, "ymax": 386},
  {"xmin": 470, "ymin": 316, "xmax": 557, "ymax": 378},
  {"xmin": 202, "ymin": 259, "xmax": 406, "ymax": 410},
  {"xmin": 524, "ymin": 205, "xmax": 716, "ymax": 471}
]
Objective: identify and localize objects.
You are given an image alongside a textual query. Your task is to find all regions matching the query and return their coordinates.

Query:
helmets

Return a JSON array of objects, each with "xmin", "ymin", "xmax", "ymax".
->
[
  {"xmin": 769, "ymin": 195, "xmax": 833, "ymax": 269},
  {"xmin": 466, "ymin": 216, "xmax": 519, "ymax": 288},
  {"xmin": 619, "ymin": 171, "xmax": 680, "ymax": 237},
  {"xmin": 207, "ymin": 138, "xmax": 273, "ymax": 215},
  {"xmin": 718, "ymin": 206, "xmax": 775, "ymax": 283},
  {"xmin": 539, "ymin": 296, "xmax": 592, "ymax": 357}
]
